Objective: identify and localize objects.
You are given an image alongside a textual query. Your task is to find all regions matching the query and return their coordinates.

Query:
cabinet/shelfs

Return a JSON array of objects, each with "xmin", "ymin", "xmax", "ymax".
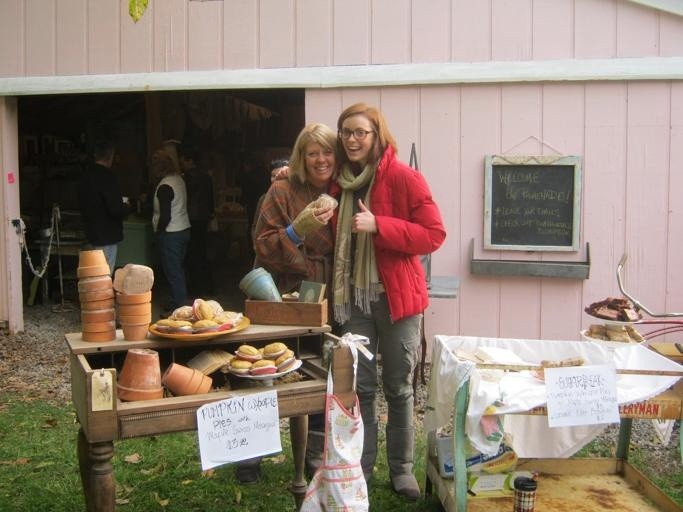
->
[
  {"xmin": 425, "ymin": 335, "xmax": 683, "ymax": 512},
  {"xmin": 114, "ymin": 213, "xmax": 154, "ymax": 268}
]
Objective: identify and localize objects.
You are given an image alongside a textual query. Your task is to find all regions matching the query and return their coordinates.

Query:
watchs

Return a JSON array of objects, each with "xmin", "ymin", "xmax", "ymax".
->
[{"xmin": 286, "ymin": 224, "xmax": 305, "ymax": 250}]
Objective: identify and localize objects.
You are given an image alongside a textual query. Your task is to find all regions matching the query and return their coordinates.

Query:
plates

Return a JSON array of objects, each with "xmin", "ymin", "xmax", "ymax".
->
[
  {"xmin": 584, "ymin": 310, "xmax": 642, "ymax": 323},
  {"xmin": 149, "ymin": 317, "xmax": 250, "ymax": 340},
  {"xmin": 580, "ymin": 330, "xmax": 646, "ymax": 345}
]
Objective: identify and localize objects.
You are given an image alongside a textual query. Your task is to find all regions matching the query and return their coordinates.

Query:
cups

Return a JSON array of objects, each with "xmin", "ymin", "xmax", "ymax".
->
[{"xmin": 514, "ymin": 477, "xmax": 537, "ymax": 512}]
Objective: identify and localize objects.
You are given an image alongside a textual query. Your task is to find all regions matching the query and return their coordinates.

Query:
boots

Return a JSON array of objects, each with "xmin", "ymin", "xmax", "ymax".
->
[
  {"xmin": 304, "ymin": 430, "xmax": 326, "ymax": 479},
  {"xmin": 386, "ymin": 423, "xmax": 421, "ymax": 501},
  {"xmin": 238, "ymin": 458, "xmax": 259, "ymax": 484},
  {"xmin": 360, "ymin": 422, "xmax": 378, "ymax": 496}
]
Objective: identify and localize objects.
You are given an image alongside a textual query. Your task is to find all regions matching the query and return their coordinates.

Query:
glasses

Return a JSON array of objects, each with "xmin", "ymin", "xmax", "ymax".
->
[{"xmin": 341, "ymin": 128, "xmax": 374, "ymax": 140}]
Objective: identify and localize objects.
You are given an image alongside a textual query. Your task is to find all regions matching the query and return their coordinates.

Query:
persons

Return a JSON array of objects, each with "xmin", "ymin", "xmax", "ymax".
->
[
  {"xmin": 275, "ymin": 105, "xmax": 447, "ymax": 499},
  {"xmin": 76, "ymin": 144, "xmax": 138, "ymax": 275},
  {"xmin": 163, "ymin": 131, "xmax": 218, "ymax": 297},
  {"xmin": 150, "ymin": 156, "xmax": 192, "ymax": 318},
  {"xmin": 236, "ymin": 123, "xmax": 337, "ymax": 483}
]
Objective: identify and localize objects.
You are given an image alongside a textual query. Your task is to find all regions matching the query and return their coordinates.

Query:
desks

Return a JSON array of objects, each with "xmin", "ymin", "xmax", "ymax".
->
[
  {"xmin": 35, "ymin": 239, "xmax": 88, "ymax": 306},
  {"xmin": 64, "ymin": 324, "xmax": 356, "ymax": 512}
]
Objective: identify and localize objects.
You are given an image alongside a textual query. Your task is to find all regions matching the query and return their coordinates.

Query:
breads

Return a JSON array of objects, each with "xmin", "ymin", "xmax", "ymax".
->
[
  {"xmin": 584, "ymin": 323, "xmax": 644, "ymax": 342},
  {"xmin": 228, "ymin": 342, "xmax": 296, "ymax": 374},
  {"xmin": 585, "ymin": 296, "xmax": 642, "ymax": 322},
  {"xmin": 155, "ymin": 299, "xmax": 239, "ymax": 333}
]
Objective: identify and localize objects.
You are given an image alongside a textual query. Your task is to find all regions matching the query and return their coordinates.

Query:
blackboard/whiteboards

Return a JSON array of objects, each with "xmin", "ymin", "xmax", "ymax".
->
[{"xmin": 483, "ymin": 154, "xmax": 582, "ymax": 253}]
